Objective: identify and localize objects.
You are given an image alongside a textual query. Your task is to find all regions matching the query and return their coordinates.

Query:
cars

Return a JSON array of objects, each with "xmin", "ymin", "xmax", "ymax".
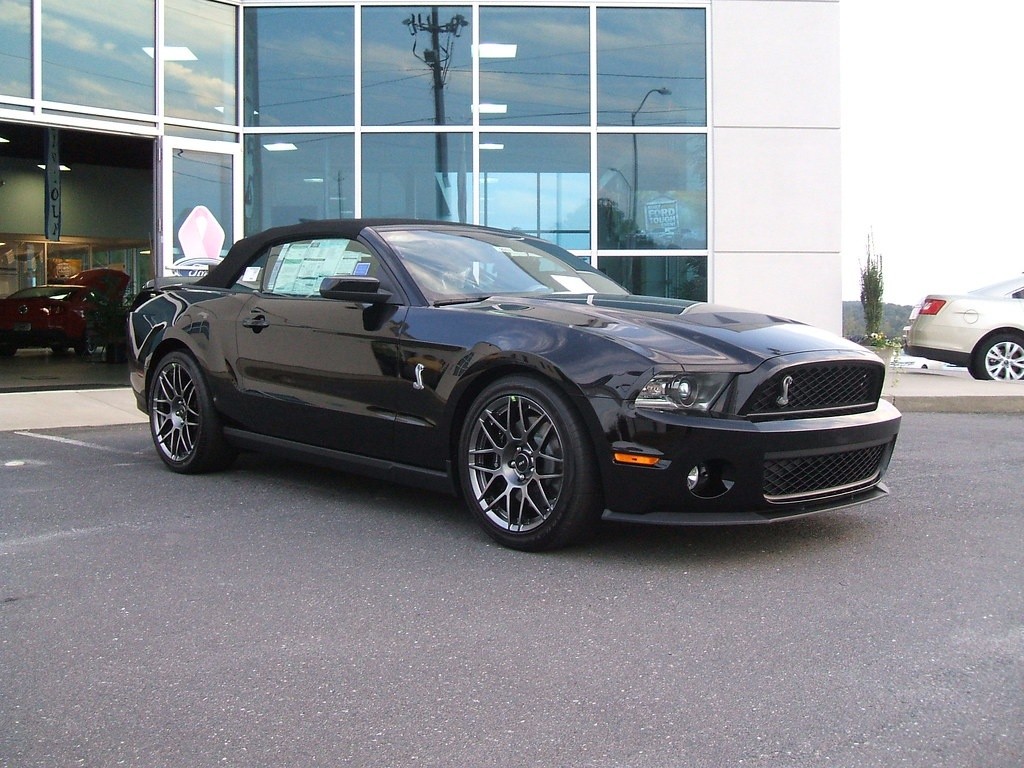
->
[
  {"xmin": 0, "ymin": 269, "xmax": 131, "ymax": 358},
  {"xmin": 123, "ymin": 217, "xmax": 903, "ymax": 553},
  {"xmin": 903, "ymin": 278, "xmax": 1024, "ymax": 381},
  {"xmin": 889, "ymin": 349, "xmax": 968, "ymax": 372}
]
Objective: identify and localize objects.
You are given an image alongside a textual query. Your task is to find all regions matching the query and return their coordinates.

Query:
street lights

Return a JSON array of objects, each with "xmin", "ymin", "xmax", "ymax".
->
[{"xmin": 631, "ymin": 87, "xmax": 675, "ymax": 293}]
